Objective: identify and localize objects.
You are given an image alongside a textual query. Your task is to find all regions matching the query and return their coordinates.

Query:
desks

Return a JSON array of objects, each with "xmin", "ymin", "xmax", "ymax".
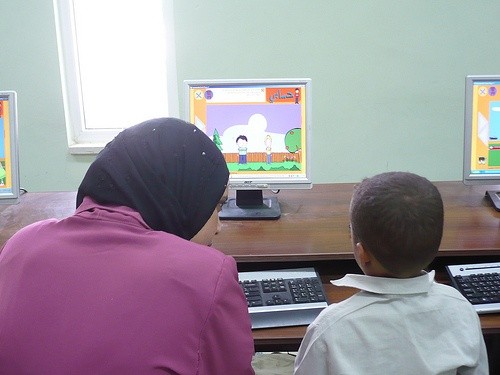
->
[{"xmin": 0, "ymin": 179, "xmax": 500, "ymax": 375}]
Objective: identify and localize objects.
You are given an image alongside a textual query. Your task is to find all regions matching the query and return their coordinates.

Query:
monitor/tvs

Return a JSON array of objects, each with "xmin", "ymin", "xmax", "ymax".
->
[
  {"xmin": 0, "ymin": 90, "xmax": 20, "ymax": 200},
  {"xmin": 464, "ymin": 75, "xmax": 500, "ymax": 209},
  {"xmin": 184, "ymin": 81, "xmax": 313, "ymax": 220}
]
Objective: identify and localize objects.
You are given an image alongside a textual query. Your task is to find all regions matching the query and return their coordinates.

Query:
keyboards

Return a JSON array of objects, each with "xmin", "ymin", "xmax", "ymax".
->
[
  {"xmin": 444, "ymin": 262, "xmax": 500, "ymax": 313},
  {"xmin": 236, "ymin": 267, "xmax": 330, "ymax": 329}
]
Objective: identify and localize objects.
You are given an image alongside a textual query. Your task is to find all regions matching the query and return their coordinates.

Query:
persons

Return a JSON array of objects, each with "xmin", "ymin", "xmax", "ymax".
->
[
  {"xmin": 0, "ymin": 118, "xmax": 257, "ymax": 375},
  {"xmin": 294, "ymin": 171, "xmax": 490, "ymax": 375}
]
